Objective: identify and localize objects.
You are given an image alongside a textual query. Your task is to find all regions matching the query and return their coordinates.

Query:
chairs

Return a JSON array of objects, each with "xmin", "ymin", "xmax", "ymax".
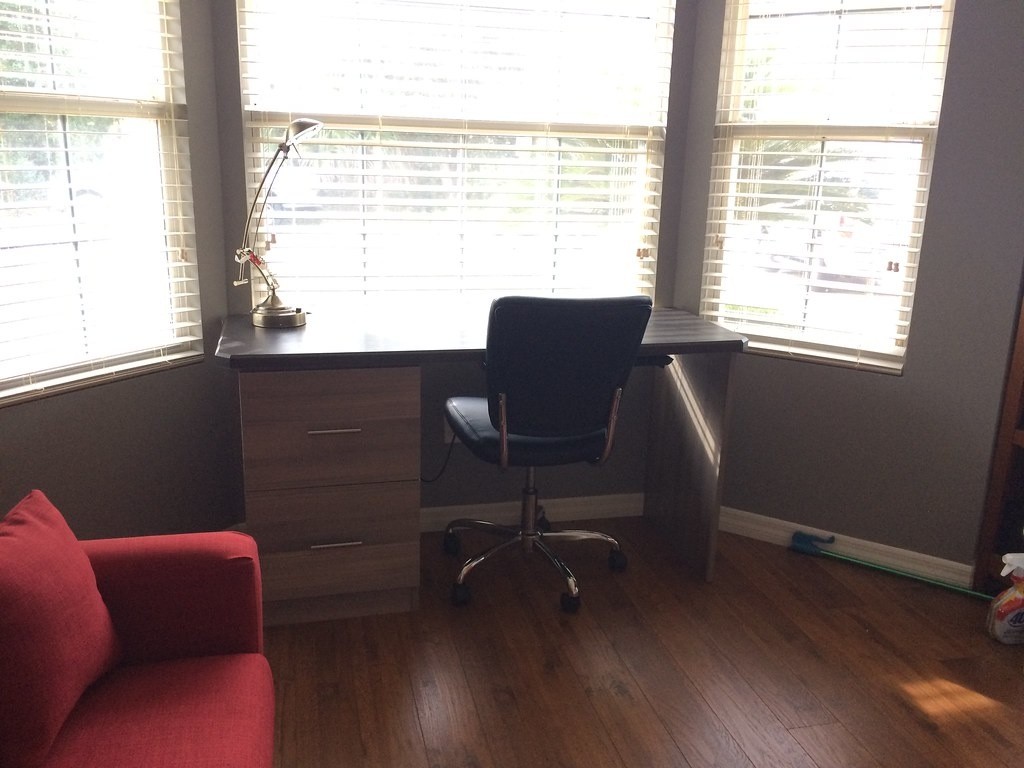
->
[{"xmin": 445, "ymin": 293, "xmax": 653, "ymax": 612}]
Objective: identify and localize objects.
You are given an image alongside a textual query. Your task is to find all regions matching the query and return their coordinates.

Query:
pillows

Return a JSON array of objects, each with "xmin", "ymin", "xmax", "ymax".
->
[{"xmin": 0, "ymin": 490, "xmax": 125, "ymax": 768}]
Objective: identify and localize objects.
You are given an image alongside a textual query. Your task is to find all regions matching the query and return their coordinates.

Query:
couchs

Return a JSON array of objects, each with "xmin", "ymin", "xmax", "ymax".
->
[{"xmin": 36, "ymin": 531, "xmax": 275, "ymax": 768}]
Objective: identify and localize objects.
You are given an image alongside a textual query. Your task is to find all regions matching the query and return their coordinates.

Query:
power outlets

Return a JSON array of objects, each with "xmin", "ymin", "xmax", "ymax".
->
[{"xmin": 444, "ymin": 415, "xmax": 461, "ymax": 444}]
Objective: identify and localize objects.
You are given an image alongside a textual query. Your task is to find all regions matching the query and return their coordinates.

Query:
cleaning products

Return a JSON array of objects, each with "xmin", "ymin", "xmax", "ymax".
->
[{"xmin": 984, "ymin": 552, "xmax": 1024, "ymax": 646}]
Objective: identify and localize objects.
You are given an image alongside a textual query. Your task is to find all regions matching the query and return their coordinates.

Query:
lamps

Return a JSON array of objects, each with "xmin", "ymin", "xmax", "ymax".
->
[{"xmin": 233, "ymin": 118, "xmax": 322, "ymax": 328}]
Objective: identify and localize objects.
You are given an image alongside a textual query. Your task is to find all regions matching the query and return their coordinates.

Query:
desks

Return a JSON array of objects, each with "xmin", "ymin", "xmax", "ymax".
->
[{"xmin": 214, "ymin": 308, "xmax": 747, "ymax": 627}]
